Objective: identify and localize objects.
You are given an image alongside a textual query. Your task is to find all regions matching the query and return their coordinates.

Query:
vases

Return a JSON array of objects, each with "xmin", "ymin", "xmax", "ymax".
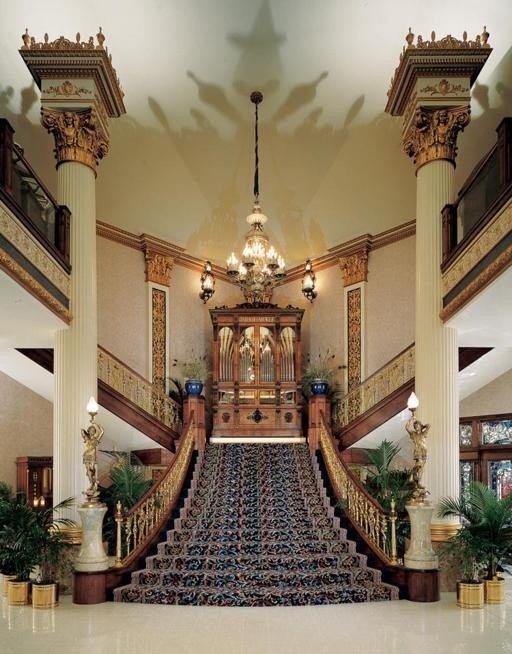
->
[
  {"xmin": 184, "ymin": 379, "xmax": 204, "ymax": 394},
  {"xmin": 311, "ymin": 379, "xmax": 329, "ymax": 393}
]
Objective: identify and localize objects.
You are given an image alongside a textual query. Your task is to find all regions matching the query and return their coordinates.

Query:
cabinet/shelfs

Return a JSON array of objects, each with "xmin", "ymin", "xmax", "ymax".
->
[{"xmin": 210, "ymin": 302, "xmax": 306, "ymax": 437}]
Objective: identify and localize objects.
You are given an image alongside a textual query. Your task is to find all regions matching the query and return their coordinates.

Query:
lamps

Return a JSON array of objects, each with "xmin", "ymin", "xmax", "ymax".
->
[
  {"xmin": 201, "ymin": 89, "xmax": 318, "ymax": 304},
  {"xmin": 404, "ymin": 391, "xmax": 432, "ymax": 506},
  {"xmin": 78, "ymin": 394, "xmax": 108, "ymax": 507}
]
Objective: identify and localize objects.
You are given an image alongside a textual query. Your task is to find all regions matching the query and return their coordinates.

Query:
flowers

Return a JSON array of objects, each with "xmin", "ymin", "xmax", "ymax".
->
[
  {"xmin": 172, "ymin": 347, "xmax": 214, "ymax": 380},
  {"xmin": 301, "ymin": 346, "xmax": 347, "ymax": 385}
]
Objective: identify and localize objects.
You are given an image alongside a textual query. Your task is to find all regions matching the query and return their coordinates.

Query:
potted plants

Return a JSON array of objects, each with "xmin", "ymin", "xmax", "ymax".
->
[
  {"xmin": 0, "ymin": 482, "xmax": 80, "ymax": 609},
  {"xmin": 434, "ymin": 479, "xmax": 511, "ymax": 608}
]
